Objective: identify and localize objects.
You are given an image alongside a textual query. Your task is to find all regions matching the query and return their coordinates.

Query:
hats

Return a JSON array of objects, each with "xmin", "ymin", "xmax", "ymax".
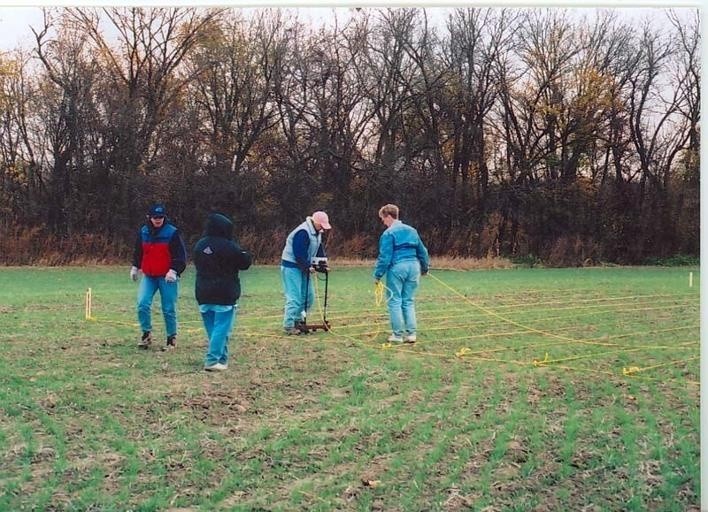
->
[
  {"xmin": 312, "ymin": 212, "xmax": 332, "ymax": 230},
  {"xmin": 149, "ymin": 206, "xmax": 166, "ymax": 217}
]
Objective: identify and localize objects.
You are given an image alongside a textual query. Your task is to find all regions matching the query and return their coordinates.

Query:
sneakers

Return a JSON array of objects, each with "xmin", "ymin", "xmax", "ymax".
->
[
  {"xmin": 389, "ymin": 335, "xmax": 404, "ymax": 342},
  {"xmin": 204, "ymin": 363, "xmax": 229, "ymax": 370},
  {"xmin": 138, "ymin": 331, "xmax": 151, "ymax": 346},
  {"xmin": 284, "ymin": 327, "xmax": 301, "ymax": 335},
  {"xmin": 163, "ymin": 337, "xmax": 176, "ymax": 351},
  {"xmin": 405, "ymin": 335, "xmax": 417, "ymax": 342}
]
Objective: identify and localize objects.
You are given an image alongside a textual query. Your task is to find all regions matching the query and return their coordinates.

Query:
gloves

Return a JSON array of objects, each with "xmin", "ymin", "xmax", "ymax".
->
[
  {"xmin": 130, "ymin": 266, "xmax": 138, "ymax": 282},
  {"xmin": 165, "ymin": 269, "xmax": 178, "ymax": 283}
]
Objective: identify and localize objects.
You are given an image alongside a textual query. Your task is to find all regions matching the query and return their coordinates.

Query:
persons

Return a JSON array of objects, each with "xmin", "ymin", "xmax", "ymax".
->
[
  {"xmin": 280, "ymin": 210, "xmax": 332, "ymax": 335},
  {"xmin": 372, "ymin": 203, "xmax": 430, "ymax": 344},
  {"xmin": 129, "ymin": 200, "xmax": 187, "ymax": 351},
  {"xmin": 192, "ymin": 212, "xmax": 253, "ymax": 371}
]
